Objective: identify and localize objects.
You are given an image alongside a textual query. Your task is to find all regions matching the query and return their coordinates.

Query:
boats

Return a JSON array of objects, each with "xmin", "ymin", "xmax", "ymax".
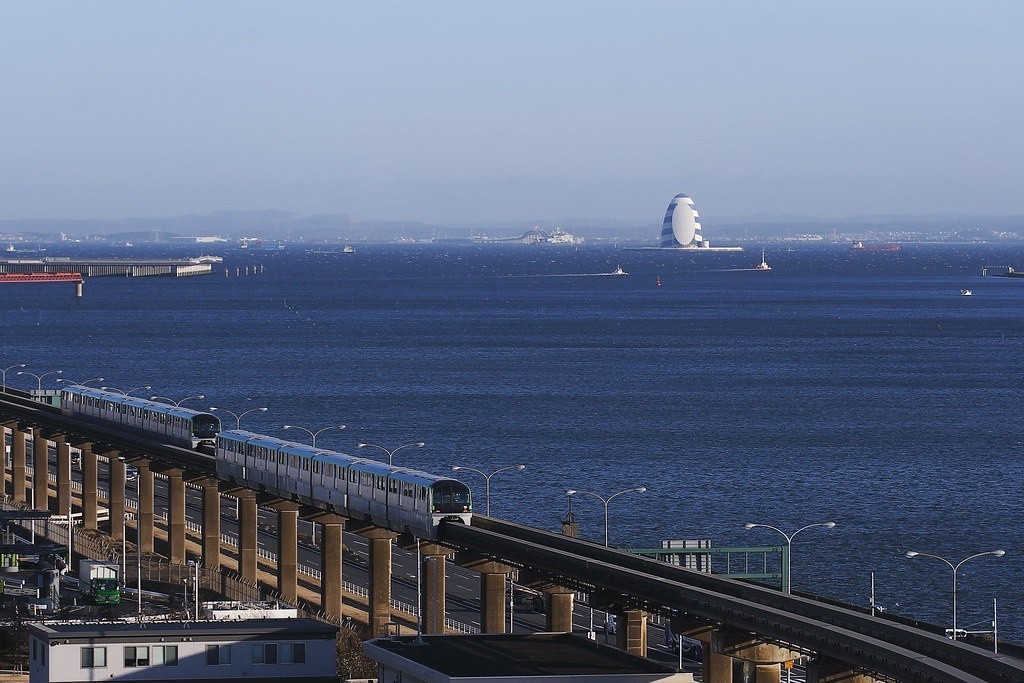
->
[
  {"xmin": 757, "ymin": 249, "xmax": 771, "ymax": 270},
  {"xmin": 613, "ymin": 265, "xmax": 628, "ymax": 275},
  {"xmin": 961, "ymin": 289, "xmax": 973, "ymax": 296}
]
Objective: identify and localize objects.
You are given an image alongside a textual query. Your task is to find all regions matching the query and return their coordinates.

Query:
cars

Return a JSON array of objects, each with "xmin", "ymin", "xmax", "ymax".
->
[{"xmin": 604, "ymin": 616, "xmax": 619, "ymax": 636}]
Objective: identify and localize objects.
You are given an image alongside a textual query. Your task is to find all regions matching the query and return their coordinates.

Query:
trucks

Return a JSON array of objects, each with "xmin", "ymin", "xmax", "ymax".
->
[{"xmin": 78, "ymin": 559, "xmax": 122, "ymax": 607}]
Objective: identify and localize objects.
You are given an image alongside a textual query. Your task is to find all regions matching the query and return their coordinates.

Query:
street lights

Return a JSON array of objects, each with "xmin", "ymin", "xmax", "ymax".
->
[
  {"xmin": 743, "ymin": 522, "xmax": 837, "ymax": 596},
  {"xmin": 15, "ymin": 370, "xmax": 62, "ymax": 403},
  {"xmin": 209, "ymin": 406, "xmax": 268, "ymax": 432},
  {"xmin": 0, "ymin": 363, "xmax": 26, "ymax": 393},
  {"xmin": 563, "ymin": 487, "xmax": 646, "ymax": 551},
  {"xmin": 905, "ymin": 549, "xmax": 1006, "ymax": 640},
  {"xmin": 282, "ymin": 424, "xmax": 346, "ymax": 447},
  {"xmin": 452, "ymin": 464, "xmax": 526, "ymax": 517},
  {"xmin": 354, "ymin": 441, "xmax": 425, "ymax": 468}
]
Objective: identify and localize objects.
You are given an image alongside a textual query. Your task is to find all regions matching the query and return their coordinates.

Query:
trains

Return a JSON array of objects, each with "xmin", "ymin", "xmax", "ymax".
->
[
  {"xmin": 59, "ymin": 386, "xmax": 222, "ymax": 451},
  {"xmin": 211, "ymin": 429, "xmax": 474, "ymax": 541}
]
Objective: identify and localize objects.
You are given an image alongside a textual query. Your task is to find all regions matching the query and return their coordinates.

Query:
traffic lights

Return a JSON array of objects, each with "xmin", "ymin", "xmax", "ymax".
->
[{"xmin": 946, "ymin": 630, "xmax": 967, "ymax": 637}]
